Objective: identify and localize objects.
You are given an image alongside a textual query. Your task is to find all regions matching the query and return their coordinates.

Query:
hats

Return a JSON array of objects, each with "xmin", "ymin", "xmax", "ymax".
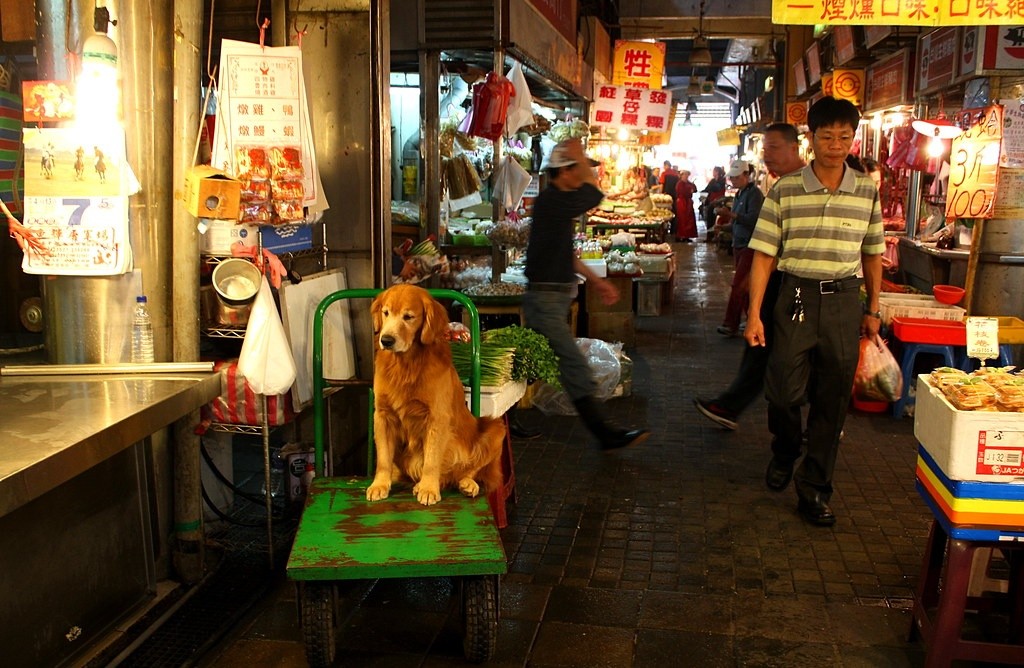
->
[
  {"xmin": 726, "ymin": 160, "xmax": 751, "ymax": 177},
  {"xmin": 540, "ymin": 144, "xmax": 602, "ymax": 172}
]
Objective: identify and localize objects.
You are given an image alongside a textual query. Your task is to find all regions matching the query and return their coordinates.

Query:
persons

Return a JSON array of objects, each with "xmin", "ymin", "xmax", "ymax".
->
[
  {"xmin": 510, "ymin": 141, "xmax": 651, "ymax": 456},
  {"xmin": 846, "ymin": 155, "xmax": 880, "ymax": 190},
  {"xmin": 691, "ymin": 123, "xmax": 843, "ymax": 442},
  {"xmin": 717, "ymin": 161, "xmax": 763, "ymax": 336},
  {"xmin": 649, "ymin": 160, "xmax": 680, "ymax": 197},
  {"xmin": 743, "ymin": 96, "xmax": 887, "ymax": 527},
  {"xmin": 675, "ymin": 171, "xmax": 698, "ymax": 243},
  {"xmin": 701, "ymin": 167, "xmax": 726, "ymax": 243}
]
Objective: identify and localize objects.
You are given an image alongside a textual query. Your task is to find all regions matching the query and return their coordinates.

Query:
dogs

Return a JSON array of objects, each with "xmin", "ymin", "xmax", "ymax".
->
[{"xmin": 367, "ymin": 283, "xmax": 508, "ymax": 506}]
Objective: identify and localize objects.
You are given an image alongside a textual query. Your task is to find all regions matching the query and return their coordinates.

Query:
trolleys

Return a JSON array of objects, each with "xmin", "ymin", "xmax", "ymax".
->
[{"xmin": 285, "ymin": 287, "xmax": 511, "ymax": 668}]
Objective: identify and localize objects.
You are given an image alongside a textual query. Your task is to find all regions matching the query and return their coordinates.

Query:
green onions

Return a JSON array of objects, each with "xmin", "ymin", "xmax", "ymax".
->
[
  {"xmin": 407, "ymin": 238, "xmax": 438, "ymax": 257},
  {"xmin": 448, "ymin": 342, "xmax": 516, "ymax": 387}
]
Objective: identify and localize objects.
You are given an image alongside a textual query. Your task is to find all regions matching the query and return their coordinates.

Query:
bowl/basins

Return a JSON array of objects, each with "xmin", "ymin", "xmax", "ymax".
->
[
  {"xmin": 934, "ymin": 285, "xmax": 965, "ymax": 304},
  {"xmin": 212, "ymin": 258, "xmax": 262, "ymax": 306}
]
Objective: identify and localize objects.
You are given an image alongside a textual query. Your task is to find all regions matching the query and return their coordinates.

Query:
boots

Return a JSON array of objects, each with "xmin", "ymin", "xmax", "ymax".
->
[
  {"xmin": 508, "ymin": 402, "xmax": 544, "ymax": 439},
  {"xmin": 570, "ymin": 395, "xmax": 650, "ymax": 451}
]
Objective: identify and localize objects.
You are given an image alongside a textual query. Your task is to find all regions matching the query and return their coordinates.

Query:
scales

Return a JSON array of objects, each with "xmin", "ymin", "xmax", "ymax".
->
[{"xmin": 926, "ymin": 201, "xmax": 946, "ymax": 234}]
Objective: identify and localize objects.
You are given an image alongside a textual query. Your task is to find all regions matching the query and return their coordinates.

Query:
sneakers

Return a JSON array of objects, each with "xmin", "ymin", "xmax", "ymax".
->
[
  {"xmin": 716, "ymin": 326, "xmax": 736, "ymax": 336},
  {"xmin": 692, "ymin": 397, "xmax": 739, "ymax": 430},
  {"xmin": 739, "ymin": 323, "xmax": 746, "ymax": 330}
]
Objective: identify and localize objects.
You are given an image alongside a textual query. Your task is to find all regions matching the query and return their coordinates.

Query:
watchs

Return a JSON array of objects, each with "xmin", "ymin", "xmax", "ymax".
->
[{"xmin": 865, "ymin": 310, "xmax": 881, "ymax": 318}]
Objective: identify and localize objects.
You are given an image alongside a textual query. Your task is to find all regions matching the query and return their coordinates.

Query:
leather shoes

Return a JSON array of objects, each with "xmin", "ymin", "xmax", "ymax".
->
[
  {"xmin": 798, "ymin": 498, "xmax": 834, "ymax": 523},
  {"xmin": 766, "ymin": 446, "xmax": 799, "ymax": 490}
]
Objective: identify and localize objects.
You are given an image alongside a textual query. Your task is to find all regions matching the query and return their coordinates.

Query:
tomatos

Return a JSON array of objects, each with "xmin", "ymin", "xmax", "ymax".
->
[{"xmin": 448, "ymin": 324, "xmax": 472, "ymax": 342}]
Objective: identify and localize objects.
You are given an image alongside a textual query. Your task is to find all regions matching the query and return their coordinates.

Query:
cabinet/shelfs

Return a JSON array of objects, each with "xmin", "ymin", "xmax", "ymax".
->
[{"xmin": 195, "ymin": 222, "xmax": 332, "ymax": 568}]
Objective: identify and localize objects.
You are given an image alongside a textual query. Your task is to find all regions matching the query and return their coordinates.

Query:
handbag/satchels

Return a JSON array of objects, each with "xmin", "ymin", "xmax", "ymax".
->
[
  {"xmin": 856, "ymin": 334, "xmax": 904, "ymax": 401},
  {"xmin": 236, "ymin": 275, "xmax": 296, "ymax": 396}
]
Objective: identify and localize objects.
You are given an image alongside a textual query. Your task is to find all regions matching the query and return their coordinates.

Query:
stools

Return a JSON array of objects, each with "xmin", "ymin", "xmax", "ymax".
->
[
  {"xmin": 486, "ymin": 413, "xmax": 517, "ymax": 528},
  {"xmin": 907, "ymin": 518, "xmax": 1024, "ymax": 668},
  {"xmin": 957, "ymin": 344, "xmax": 1013, "ymax": 373},
  {"xmin": 895, "ymin": 345, "xmax": 954, "ymax": 416}
]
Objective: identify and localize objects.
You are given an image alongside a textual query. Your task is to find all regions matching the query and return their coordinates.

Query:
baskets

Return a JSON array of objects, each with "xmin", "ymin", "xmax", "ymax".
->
[{"xmin": 860, "ymin": 283, "xmax": 965, "ymax": 326}]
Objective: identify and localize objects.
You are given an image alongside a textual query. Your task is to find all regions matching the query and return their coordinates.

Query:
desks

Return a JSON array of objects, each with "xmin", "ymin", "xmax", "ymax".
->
[
  {"xmin": 462, "ymin": 300, "xmax": 578, "ymax": 338},
  {"xmin": 593, "ymin": 223, "xmax": 664, "ymax": 243}
]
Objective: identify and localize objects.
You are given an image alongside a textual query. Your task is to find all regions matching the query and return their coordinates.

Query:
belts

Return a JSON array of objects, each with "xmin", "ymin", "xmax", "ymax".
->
[
  {"xmin": 525, "ymin": 283, "xmax": 572, "ymax": 293},
  {"xmin": 782, "ymin": 274, "xmax": 866, "ymax": 294}
]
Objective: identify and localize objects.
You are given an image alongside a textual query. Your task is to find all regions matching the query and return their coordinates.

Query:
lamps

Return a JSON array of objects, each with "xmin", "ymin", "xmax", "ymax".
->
[
  {"xmin": 684, "ymin": 36, "xmax": 712, "ymax": 125},
  {"xmin": 913, "ymin": 97, "xmax": 963, "ymax": 156}
]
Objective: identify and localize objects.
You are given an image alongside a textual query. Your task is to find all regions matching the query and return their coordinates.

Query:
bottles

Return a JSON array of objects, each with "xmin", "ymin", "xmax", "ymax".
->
[
  {"xmin": 261, "ymin": 448, "xmax": 284, "ymax": 496},
  {"xmin": 581, "ymin": 239, "xmax": 603, "ymax": 259},
  {"xmin": 131, "ymin": 296, "xmax": 154, "ymax": 364}
]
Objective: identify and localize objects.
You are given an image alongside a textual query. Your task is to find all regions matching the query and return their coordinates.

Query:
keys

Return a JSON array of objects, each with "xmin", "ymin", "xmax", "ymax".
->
[{"xmin": 791, "ymin": 298, "xmax": 804, "ymax": 322}]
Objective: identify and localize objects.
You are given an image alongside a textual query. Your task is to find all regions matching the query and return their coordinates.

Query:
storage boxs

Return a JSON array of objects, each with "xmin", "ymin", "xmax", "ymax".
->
[
  {"xmin": 464, "ymin": 378, "xmax": 526, "ymax": 418},
  {"xmin": 860, "ymin": 285, "xmax": 1024, "ymax": 542},
  {"xmin": 580, "ymin": 253, "xmax": 673, "ymax": 350},
  {"xmin": 184, "ymin": 164, "xmax": 312, "ymax": 254}
]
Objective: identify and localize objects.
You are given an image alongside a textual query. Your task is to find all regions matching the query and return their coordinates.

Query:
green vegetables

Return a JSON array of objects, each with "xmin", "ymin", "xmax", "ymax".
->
[{"xmin": 482, "ymin": 323, "xmax": 564, "ymax": 387}]
